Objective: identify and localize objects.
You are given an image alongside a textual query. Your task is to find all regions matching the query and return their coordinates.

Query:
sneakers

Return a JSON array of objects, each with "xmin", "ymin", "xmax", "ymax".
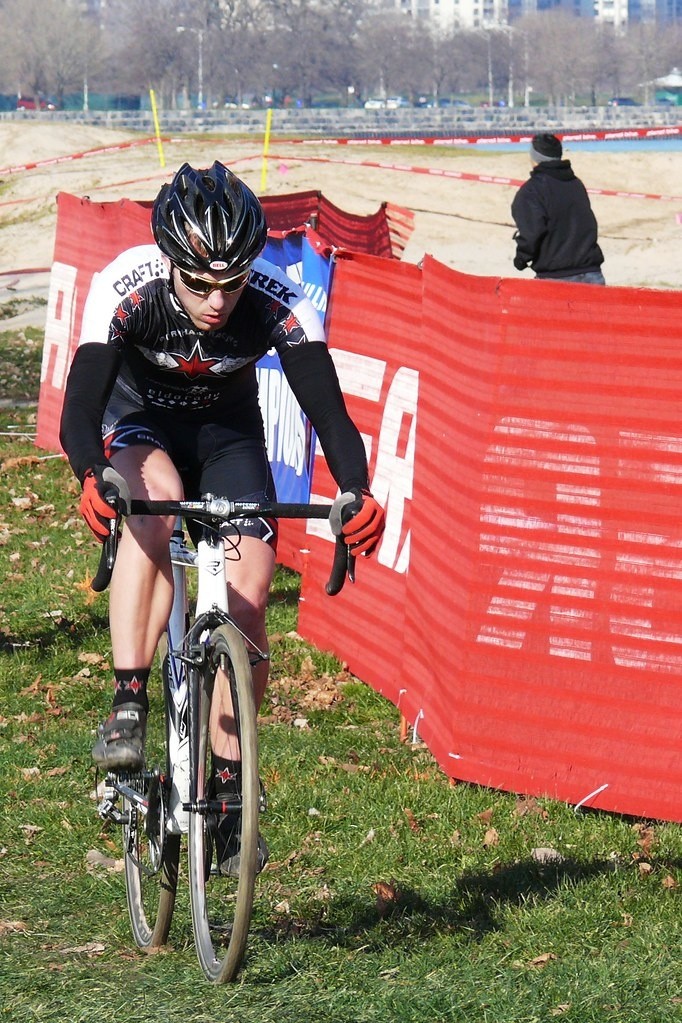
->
[
  {"xmin": 92, "ymin": 702, "xmax": 146, "ymax": 772},
  {"xmin": 204, "ymin": 777, "xmax": 269, "ymax": 879}
]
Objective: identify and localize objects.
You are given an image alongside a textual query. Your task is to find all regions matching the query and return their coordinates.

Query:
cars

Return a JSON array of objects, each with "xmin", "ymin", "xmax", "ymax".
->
[
  {"xmin": 211, "ymin": 89, "xmax": 303, "ymax": 110},
  {"xmin": 422, "ymin": 98, "xmax": 468, "ymax": 109},
  {"xmin": 607, "ymin": 98, "xmax": 642, "ymax": 107},
  {"xmin": 480, "ymin": 100, "xmax": 509, "ymax": 107},
  {"xmin": 364, "ymin": 96, "xmax": 412, "ymax": 110},
  {"xmin": 19, "ymin": 96, "xmax": 58, "ymax": 111}
]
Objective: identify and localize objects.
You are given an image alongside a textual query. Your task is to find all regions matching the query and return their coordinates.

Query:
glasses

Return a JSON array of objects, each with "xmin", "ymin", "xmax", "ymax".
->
[{"xmin": 170, "ymin": 260, "xmax": 251, "ymax": 295}]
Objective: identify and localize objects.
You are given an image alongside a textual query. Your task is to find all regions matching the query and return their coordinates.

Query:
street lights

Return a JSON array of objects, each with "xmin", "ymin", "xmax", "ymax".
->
[{"xmin": 175, "ymin": 26, "xmax": 207, "ymax": 110}]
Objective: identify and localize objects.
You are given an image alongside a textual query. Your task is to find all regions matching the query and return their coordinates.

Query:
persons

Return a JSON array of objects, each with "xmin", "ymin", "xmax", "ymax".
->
[
  {"xmin": 61, "ymin": 161, "xmax": 385, "ymax": 881},
  {"xmin": 512, "ymin": 134, "xmax": 605, "ymax": 285}
]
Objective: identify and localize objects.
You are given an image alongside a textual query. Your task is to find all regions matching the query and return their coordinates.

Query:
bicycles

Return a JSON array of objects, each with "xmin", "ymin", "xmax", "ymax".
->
[{"xmin": 80, "ymin": 480, "xmax": 368, "ymax": 981}]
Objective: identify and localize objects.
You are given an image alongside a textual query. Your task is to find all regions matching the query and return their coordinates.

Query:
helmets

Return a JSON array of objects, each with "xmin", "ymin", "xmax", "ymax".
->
[{"xmin": 152, "ymin": 159, "xmax": 267, "ymax": 273}]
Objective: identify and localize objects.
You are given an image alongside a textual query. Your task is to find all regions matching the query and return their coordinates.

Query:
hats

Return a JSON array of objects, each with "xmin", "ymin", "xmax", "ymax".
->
[{"xmin": 529, "ymin": 132, "xmax": 563, "ymax": 163}]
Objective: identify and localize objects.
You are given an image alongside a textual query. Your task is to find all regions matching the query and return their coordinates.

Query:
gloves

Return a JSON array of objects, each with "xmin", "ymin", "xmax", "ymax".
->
[
  {"xmin": 79, "ymin": 463, "xmax": 132, "ymax": 543},
  {"xmin": 515, "ymin": 256, "xmax": 529, "ymax": 271},
  {"xmin": 329, "ymin": 486, "xmax": 386, "ymax": 559}
]
